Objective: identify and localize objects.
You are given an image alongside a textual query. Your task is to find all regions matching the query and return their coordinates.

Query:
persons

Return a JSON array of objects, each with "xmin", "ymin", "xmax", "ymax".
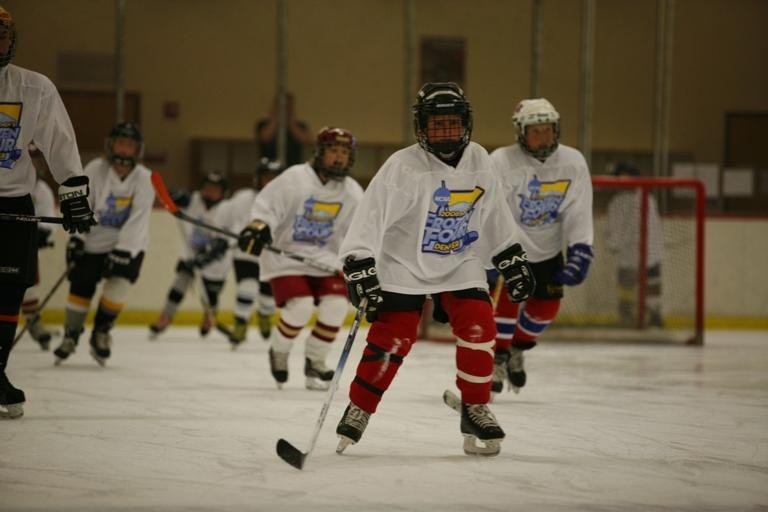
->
[
  {"xmin": 1, "ymin": 7, "xmax": 101, "ymax": 406},
  {"xmin": 487, "ymin": 94, "xmax": 594, "ymax": 400},
  {"xmin": 256, "ymin": 92, "xmax": 313, "ymax": 185},
  {"xmin": 21, "ymin": 140, "xmax": 53, "ymax": 350},
  {"xmin": 239, "ymin": 128, "xmax": 362, "ymax": 389},
  {"xmin": 603, "ymin": 160, "xmax": 662, "ymax": 327},
  {"xmin": 149, "ymin": 159, "xmax": 283, "ymax": 349},
  {"xmin": 52, "ymin": 123, "xmax": 156, "ymax": 365},
  {"xmin": 335, "ymin": 81, "xmax": 535, "ymax": 441}
]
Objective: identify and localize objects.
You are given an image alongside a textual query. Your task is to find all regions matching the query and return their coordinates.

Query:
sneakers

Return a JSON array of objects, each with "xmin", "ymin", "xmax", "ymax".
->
[
  {"xmin": 54, "ymin": 326, "xmax": 84, "ymax": 366},
  {"xmin": 269, "ymin": 348, "xmax": 289, "ymax": 389},
  {"xmin": 230, "ymin": 317, "xmax": 248, "ymax": 351},
  {"xmin": 90, "ymin": 330, "xmax": 111, "ymax": 367},
  {"xmin": 337, "ymin": 402, "xmax": 370, "ymax": 442},
  {"xmin": 0, "ymin": 372, "xmax": 26, "ymax": 418},
  {"xmin": 259, "ymin": 315, "xmax": 271, "ymax": 339},
  {"xmin": 460, "ymin": 349, "xmax": 527, "ymax": 439},
  {"xmin": 200, "ymin": 318, "xmax": 211, "ymax": 335},
  {"xmin": 26, "ymin": 318, "xmax": 53, "ymax": 350},
  {"xmin": 305, "ymin": 355, "xmax": 334, "ymax": 391},
  {"xmin": 150, "ymin": 315, "xmax": 172, "ymax": 340}
]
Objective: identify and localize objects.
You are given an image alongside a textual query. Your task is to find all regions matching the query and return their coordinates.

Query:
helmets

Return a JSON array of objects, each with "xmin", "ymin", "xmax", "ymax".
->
[
  {"xmin": 414, "ymin": 81, "xmax": 472, "ymax": 155},
  {"xmin": 104, "ymin": 121, "xmax": 354, "ymax": 191},
  {"xmin": 513, "ymin": 98, "xmax": 562, "ymax": 157},
  {"xmin": 0, "ymin": 9, "xmax": 16, "ymax": 67}
]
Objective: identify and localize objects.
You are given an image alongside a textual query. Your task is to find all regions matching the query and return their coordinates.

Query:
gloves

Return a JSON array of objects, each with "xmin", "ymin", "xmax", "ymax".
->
[
  {"xmin": 561, "ymin": 243, "xmax": 592, "ymax": 286},
  {"xmin": 341, "ymin": 258, "xmax": 383, "ymax": 310},
  {"xmin": 491, "ymin": 242, "xmax": 535, "ymax": 302},
  {"xmin": 57, "ymin": 176, "xmax": 268, "ymax": 277}
]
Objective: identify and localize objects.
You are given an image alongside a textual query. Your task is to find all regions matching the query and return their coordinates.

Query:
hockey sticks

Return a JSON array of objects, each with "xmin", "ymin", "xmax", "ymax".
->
[
  {"xmin": 276, "ymin": 298, "xmax": 369, "ymax": 469},
  {"xmin": 151, "ymin": 171, "xmax": 345, "ymax": 278}
]
